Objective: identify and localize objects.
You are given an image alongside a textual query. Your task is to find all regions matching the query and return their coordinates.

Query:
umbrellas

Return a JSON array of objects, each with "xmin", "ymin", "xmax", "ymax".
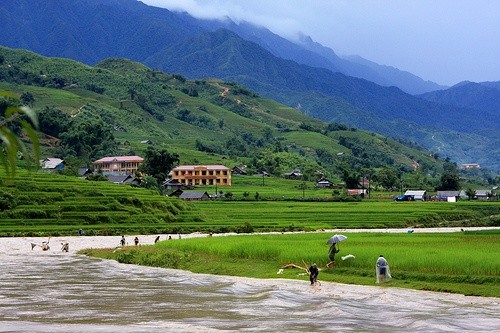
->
[{"xmin": 325, "ymin": 234, "xmax": 348, "ymax": 245}]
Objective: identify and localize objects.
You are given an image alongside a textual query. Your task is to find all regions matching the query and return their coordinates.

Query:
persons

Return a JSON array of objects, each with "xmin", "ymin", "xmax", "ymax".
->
[
  {"xmin": 376, "ymin": 255, "xmax": 392, "ymax": 284},
  {"xmin": 326, "ymin": 243, "xmax": 340, "ymax": 268},
  {"xmin": 178, "ymin": 233, "xmax": 182, "ymax": 238},
  {"xmin": 408, "ymin": 230, "xmax": 414, "ymax": 233},
  {"xmin": 155, "ymin": 236, "xmax": 160, "ymax": 242},
  {"xmin": 121, "ymin": 235, "xmax": 126, "ymax": 246},
  {"xmin": 168, "ymin": 236, "xmax": 172, "ymax": 239},
  {"xmin": 209, "ymin": 231, "xmax": 213, "ymax": 236},
  {"xmin": 40, "ymin": 241, "xmax": 70, "ymax": 252},
  {"xmin": 308, "ymin": 263, "xmax": 319, "ymax": 286},
  {"xmin": 78, "ymin": 229, "xmax": 84, "ymax": 236},
  {"xmin": 134, "ymin": 236, "xmax": 139, "ymax": 245}
]
